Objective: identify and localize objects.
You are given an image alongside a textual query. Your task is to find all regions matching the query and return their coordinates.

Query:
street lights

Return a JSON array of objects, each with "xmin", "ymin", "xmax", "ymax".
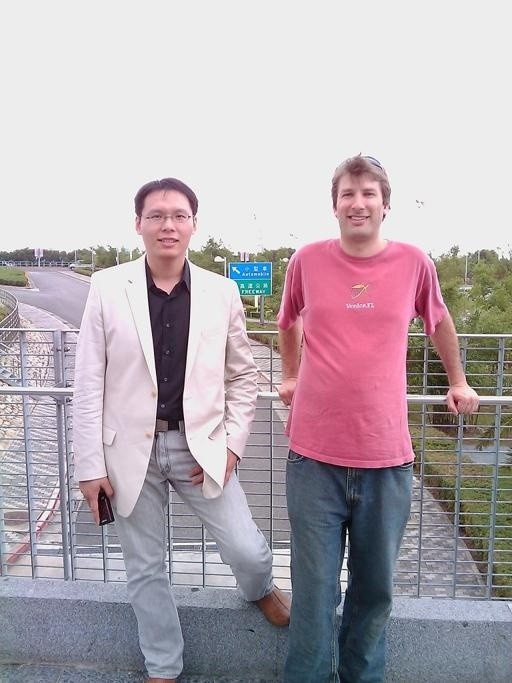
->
[{"xmin": 214, "ymin": 256, "xmax": 226, "ymax": 276}]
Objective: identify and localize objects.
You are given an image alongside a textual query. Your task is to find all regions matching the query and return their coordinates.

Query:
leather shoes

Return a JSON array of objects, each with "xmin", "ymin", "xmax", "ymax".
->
[
  {"xmin": 146, "ymin": 678, "xmax": 176, "ymax": 683},
  {"xmin": 255, "ymin": 584, "xmax": 291, "ymax": 627}
]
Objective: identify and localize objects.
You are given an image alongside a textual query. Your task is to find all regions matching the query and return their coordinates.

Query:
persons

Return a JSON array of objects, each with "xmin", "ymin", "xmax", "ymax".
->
[
  {"xmin": 277, "ymin": 156, "xmax": 479, "ymax": 683},
  {"xmin": 72, "ymin": 178, "xmax": 291, "ymax": 683}
]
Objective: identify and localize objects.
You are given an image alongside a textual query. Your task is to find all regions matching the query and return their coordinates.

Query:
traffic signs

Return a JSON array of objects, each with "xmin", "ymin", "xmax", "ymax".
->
[{"xmin": 229, "ymin": 262, "xmax": 272, "ymax": 296}]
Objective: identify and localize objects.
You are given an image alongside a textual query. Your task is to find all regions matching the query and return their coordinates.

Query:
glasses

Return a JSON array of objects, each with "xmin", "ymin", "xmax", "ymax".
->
[{"xmin": 141, "ymin": 214, "xmax": 194, "ymax": 223}]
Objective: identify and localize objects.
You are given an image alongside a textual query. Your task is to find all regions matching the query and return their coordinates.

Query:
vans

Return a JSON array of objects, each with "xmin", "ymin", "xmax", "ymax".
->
[{"xmin": 69, "ymin": 260, "xmax": 95, "ymax": 271}]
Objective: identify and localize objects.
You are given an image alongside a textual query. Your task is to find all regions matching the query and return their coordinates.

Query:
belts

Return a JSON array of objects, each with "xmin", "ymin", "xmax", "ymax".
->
[{"xmin": 155, "ymin": 420, "xmax": 185, "ymax": 432}]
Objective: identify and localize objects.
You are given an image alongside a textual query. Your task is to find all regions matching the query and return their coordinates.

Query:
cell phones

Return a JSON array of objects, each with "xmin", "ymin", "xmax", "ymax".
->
[{"xmin": 98, "ymin": 488, "xmax": 115, "ymax": 525}]
